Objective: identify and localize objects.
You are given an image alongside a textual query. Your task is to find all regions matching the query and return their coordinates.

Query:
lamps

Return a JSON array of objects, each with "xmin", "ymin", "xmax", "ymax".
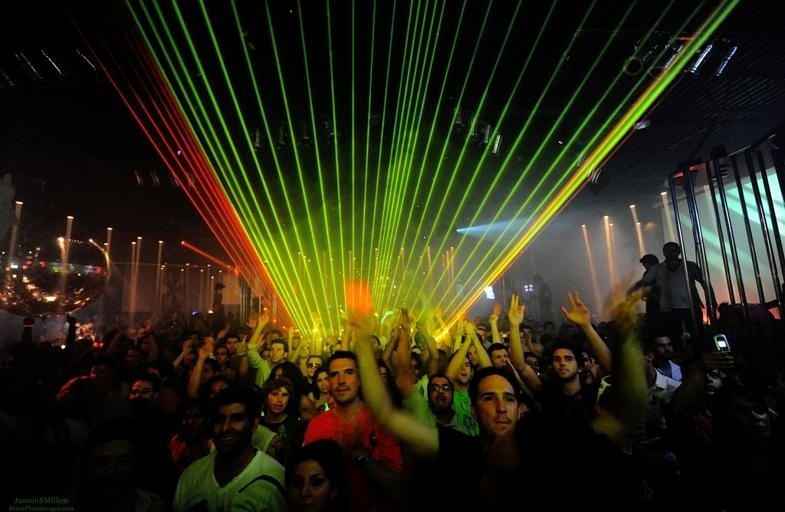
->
[
  {"xmin": 622, "ymin": 30, "xmax": 740, "ymax": 85},
  {"xmin": 246, "ymin": 110, "xmax": 342, "ymax": 155},
  {"xmin": 450, "ymin": 95, "xmax": 504, "ymax": 157}
]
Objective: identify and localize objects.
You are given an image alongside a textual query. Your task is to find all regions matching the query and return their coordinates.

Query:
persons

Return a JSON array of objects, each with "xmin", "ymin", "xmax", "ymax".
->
[
  {"xmin": 639, "ymin": 252, "xmax": 662, "ymax": 316},
  {"xmin": 532, "ymin": 274, "xmax": 555, "ymax": 320},
  {"xmin": 1, "ymin": 287, "xmax": 784, "ymax": 512},
  {"xmin": 213, "ymin": 282, "xmax": 226, "ymax": 320},
  {"xmin": 627, "ymin": 240, "xmax": 719, "ymax": 339}
]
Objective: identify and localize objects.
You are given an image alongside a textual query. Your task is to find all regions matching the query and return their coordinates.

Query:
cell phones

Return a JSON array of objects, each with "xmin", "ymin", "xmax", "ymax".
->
[{"xmin": 712, "ymin": 334, "xmax": 732, "ymax": 353}]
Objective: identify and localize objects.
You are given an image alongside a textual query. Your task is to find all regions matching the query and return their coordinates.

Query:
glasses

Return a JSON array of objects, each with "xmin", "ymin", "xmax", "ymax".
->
[{"xmin": 431, "ymin": 383, "xmax": 450, "ymax": 391}]
